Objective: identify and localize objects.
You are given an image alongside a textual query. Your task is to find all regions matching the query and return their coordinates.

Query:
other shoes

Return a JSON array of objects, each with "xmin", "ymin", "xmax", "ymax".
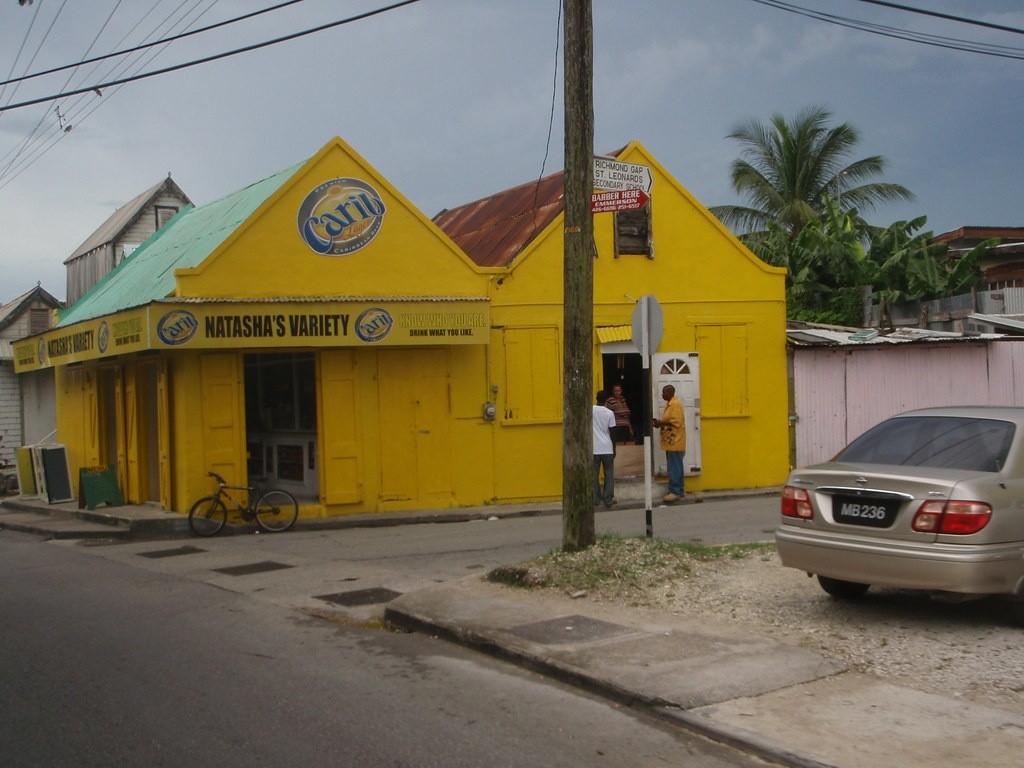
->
[
  {"xmin": 606, "ymin": 499, "xmax": 617, "ymax": 507},
  {"xmin": 592, "ymin": 501, "xmax": 601, "ymax": 506},
  {"xmin": 663, "ymin": 493, "xmax": 685, "ymax": 502}
]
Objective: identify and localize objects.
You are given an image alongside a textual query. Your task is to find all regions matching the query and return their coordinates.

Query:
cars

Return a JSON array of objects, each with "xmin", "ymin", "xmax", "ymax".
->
[{"xmin": 775, "ymin": 405, "xmax": 1024, "ymax": 629}]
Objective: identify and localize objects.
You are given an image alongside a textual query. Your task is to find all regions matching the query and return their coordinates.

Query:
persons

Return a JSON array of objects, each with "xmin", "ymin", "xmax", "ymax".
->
[
  {"xmin": 605, "ymin": 384, "xmax": 634, "ymax": 443},
  {"xmin": 653, "ymin": 385, "xmax": 687, "ymax": 502},
  {"xmin": 593, "ymin": 391, "xmax": 616, "ymax": 507}
]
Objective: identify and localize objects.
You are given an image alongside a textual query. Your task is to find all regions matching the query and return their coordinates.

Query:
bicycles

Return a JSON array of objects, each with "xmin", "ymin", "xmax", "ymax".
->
[{"xmin": 189, "ymin": 471, "xmax": 298, "ymax": 537}]
